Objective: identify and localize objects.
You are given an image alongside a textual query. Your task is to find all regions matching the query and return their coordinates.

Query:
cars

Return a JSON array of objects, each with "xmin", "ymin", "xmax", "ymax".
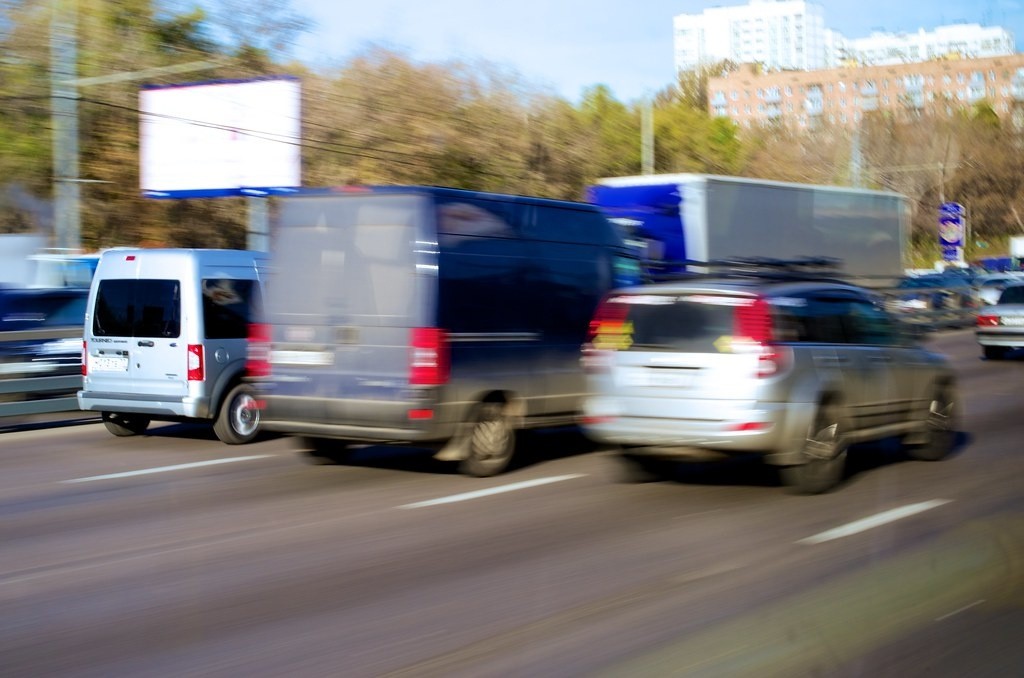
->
[
  {"xmin": 78, "ymin": 247, "xmax": 268, "ymax": 445},
  {"xmin": 900, "ymin": 271, "xmax": 1020, "ymax": 320},
  {"xmin": 973, "ymin": 281, "xmax": 1024, "ymax": 359}
]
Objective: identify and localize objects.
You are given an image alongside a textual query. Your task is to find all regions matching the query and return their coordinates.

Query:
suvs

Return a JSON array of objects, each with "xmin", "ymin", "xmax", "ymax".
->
[{"xmin": 579, "ymin": 258, "xmax": 960, "ymax": 495}]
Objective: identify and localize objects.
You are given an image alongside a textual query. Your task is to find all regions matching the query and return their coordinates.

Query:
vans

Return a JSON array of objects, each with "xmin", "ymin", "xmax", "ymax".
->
[{"xmin": 248, "ymin": 182, "xmax": 654, "ymax": 478}]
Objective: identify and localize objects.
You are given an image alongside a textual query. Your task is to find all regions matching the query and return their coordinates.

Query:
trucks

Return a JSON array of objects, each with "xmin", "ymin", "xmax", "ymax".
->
[{"xmin": 589, "ymin": 172, "xmax": 908, "ymax": 288}]
